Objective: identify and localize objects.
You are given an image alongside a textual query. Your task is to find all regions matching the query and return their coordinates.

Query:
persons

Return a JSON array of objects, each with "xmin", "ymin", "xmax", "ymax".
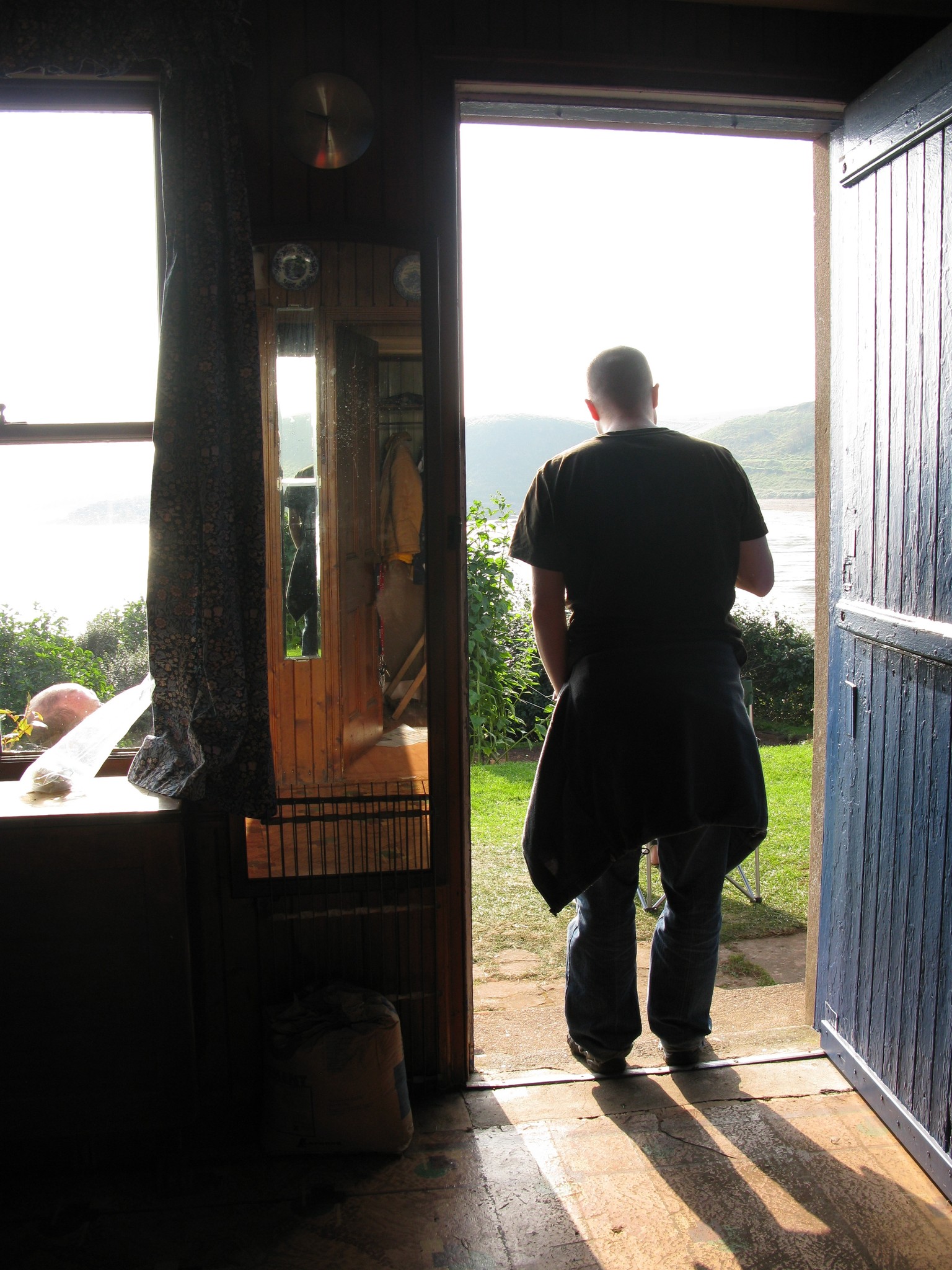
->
[
  {"xmin": 510, "ymin": 344, "xmax": 776, "ymax": 1073},
  {"xmin": 282, "ymin": 464, "xmax": 318, "ymax": 655}
]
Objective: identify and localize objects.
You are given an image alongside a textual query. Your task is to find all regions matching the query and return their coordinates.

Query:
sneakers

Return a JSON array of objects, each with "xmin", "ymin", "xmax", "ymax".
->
[
  {"xmin": 568, "ymin": 1033, "xmax": 627, "ymax": 1076},
  {"xmin": 659, "ymin": 1036, "xmax": 706, "ymax": 1068}
]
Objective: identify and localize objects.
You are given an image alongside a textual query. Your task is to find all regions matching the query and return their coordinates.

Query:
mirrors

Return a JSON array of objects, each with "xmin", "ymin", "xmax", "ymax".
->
[{"xmin": 242, "ymin": 220, "xmax": 440, "ymax": 884}]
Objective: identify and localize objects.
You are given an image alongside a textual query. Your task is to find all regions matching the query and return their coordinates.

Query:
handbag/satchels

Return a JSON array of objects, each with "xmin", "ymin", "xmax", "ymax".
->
[{"xmin": 14, "ymin": 675, "xmax": 155, "ymax": 807}]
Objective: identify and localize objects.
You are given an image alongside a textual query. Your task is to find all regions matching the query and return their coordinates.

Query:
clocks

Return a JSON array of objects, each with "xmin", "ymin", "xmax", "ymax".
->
[{"xmin": 278, "ymin": 72, "xmax": 373, "ymax": 169}]
